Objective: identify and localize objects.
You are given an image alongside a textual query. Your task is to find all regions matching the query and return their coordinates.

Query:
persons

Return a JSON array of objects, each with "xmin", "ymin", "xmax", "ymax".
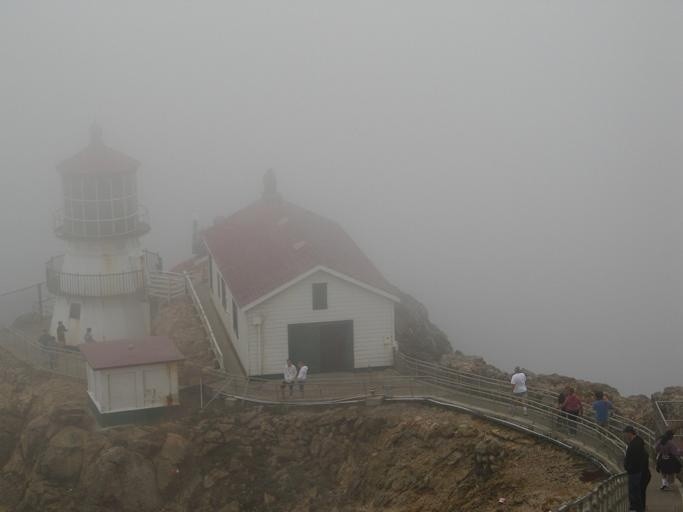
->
[
  {"xmin": 558, "ymin": 389, "xmax": 584, "ymax": 431},
  {"xmin": 55, "ymin": 320, "xmax": 69, "ymax": 345},
  {"xmin": 652, "ymin": 429, "xmax": 682, "ymax": 491},
  {"xmin": 82, "ymin": 327, "xmax": 96, "ymax": 343},
  {"xmin": 277, "ymin": 357, "xmax": 297, "ymax": 397},
  {"xmin": 45, "ymin": 336, "xmax": 60, "ymax": 370},
  {"xmin": 294, "ymin": 360, "xmax": 309, "ymax": 400},
  {"xmin": 38, "ymin": 329, "xmax": 52, "ymax": 356},
  {"xmin": 590, "ymin": 391, "xmax": 614, "ymax": 440},
  {"xmin": 555, "ymin": 385, "xmax": 573, "ymax": 430},
  {"xmin": 508, "ymin": 366, "xmax": 529, "ymax": 417},
  {"xmin": 620, "ymin": 425, "xmax": 651, "ymax": 511}
]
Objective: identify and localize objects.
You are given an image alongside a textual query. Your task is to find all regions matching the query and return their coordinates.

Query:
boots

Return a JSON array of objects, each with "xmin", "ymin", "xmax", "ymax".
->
[{"xmin": 660, "ymin": 478, "xmax": 667, "ymax": 489}]
